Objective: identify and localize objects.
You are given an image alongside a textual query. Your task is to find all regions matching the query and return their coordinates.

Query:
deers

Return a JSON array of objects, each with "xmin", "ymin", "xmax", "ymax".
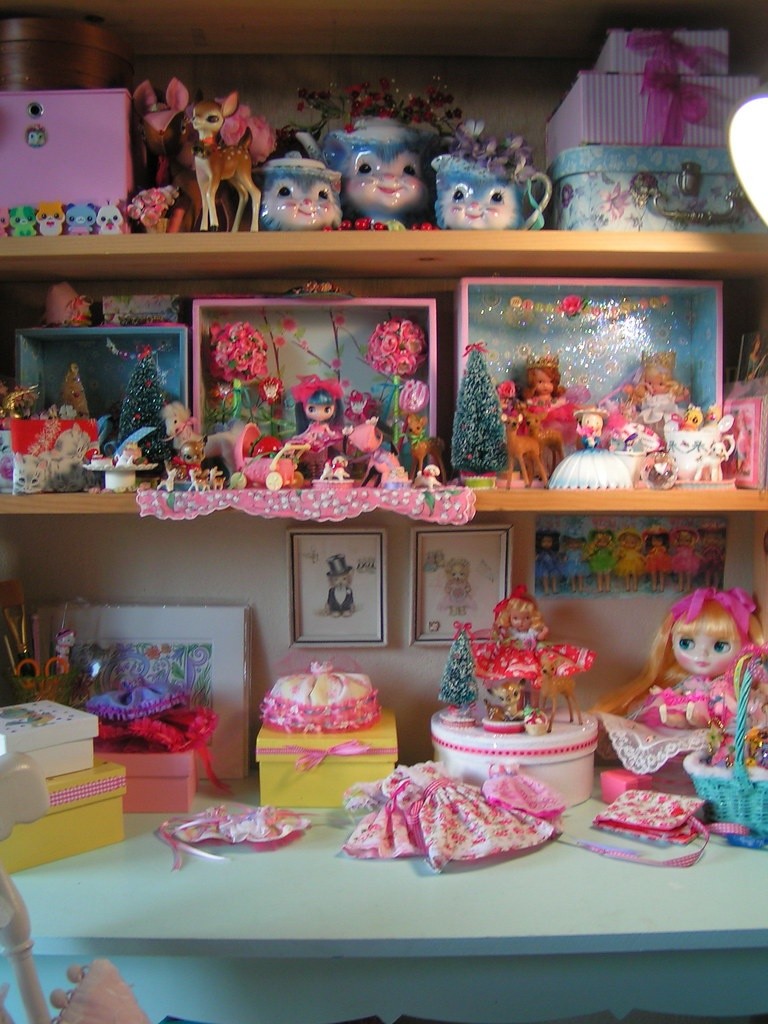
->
[
  {"xmin": 483, "ymin": 679, "xmax": 526, "ymax": 721},
  {"xmin": 190, "ymin": 89, "xmax": 261, "ymax": 232},
  {"xmin": 405, "ymin": 415, "xmax": 448, "ymax": 487},
  {"xmin": 501, "ymin": 410, "xmax": 565, "ymax": 490},
  {"xmin": 539, "ymin": 653, "xmax": 584, "ymax": 733}
]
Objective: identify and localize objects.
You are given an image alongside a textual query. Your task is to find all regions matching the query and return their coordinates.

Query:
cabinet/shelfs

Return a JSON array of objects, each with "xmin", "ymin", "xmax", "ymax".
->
[{"xmin": 0, "ymin": 0, "xmax": 768, "ymax": 1014}]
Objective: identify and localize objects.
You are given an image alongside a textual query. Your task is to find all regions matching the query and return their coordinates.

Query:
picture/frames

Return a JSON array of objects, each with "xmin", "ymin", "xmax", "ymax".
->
[
  {"xmin": 285, "ymin": 525, "xmax": 389, "ymax": 647},
  {"xmin": 407, "ymin": 523, "xmax": 514, "ymax": 646}
]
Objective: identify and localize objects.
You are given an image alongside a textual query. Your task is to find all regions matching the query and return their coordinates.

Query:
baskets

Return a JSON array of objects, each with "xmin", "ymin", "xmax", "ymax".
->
[{"xmin": 683, "ymin": 657, "xmax": 768, "ymax": 833}]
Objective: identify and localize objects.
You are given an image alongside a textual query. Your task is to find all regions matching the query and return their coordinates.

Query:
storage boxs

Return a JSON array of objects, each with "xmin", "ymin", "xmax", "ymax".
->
[
  {"xmin": 431, "ymin": 704, "xmax": 599, "ymax": 812},
  {"xmin": 544, "ymin": 30, "xmax": 766, "ymax": 230},
  {"xmin": 97, "ymin": 736, "xmax": 197, "ymax": 815},
  {"xmin": 0, "ymin": 699, "xmax": 125, "ymax": 877},
  {"xmin": 257, "ymin": 704, "xmax": 399, "ymax": 815},
  {"xmin": 16, "ymin": 276, "xmax": 723, "ymax": 488}
]
